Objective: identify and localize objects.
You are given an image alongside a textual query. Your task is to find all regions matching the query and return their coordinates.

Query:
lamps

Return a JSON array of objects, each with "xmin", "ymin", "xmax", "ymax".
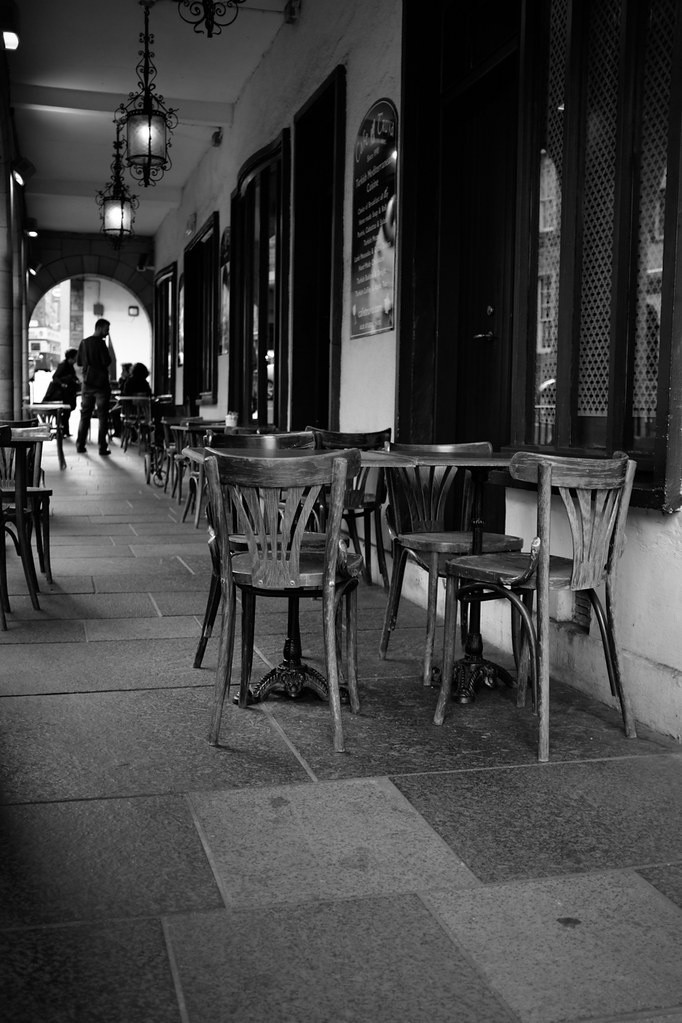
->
[
  {"xmin": 28, "ymin": 259, "xmax": 44, "ymax": 276},
  {"xmin": 113, "ymin": 0, "xmax": 179, "ymax": 186},
  {"xmin": 23, "ymin": 217, "xmax": 39, "ymax": 237},
  {"xmin": 13, "ymin": 158, "xmax": 37, "ymax": 187},
  {"xmin": 95, "ymin": 119, "xmax": 140, "ymax": 250}
]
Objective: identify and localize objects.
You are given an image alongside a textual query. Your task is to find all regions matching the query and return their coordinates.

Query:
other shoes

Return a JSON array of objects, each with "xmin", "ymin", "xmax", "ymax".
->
[
  {"xmin": 77, "ymin": 447, "xmax": 86, "ymax": 452},
  {"xmin": 111, "ymin": 432, "xmax": 121, "ymax": 437},
  {"xmin": 131, "ymin": 434, "xmax": 138, "ymax": 442},
  {"xmin": 99, "ymin": 450, "xmax": 110, "ymax": 455}
]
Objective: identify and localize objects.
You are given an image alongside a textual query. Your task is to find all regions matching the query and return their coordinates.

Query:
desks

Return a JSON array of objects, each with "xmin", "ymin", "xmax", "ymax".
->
[
  {"xmin": 378, "ymin": 448, "xmax": 525, "ymax": 709},
  {"xmin": 171, "ymin": 424, "xmax": 273, "ymax": 502},
  {"xmin": 184, "ymin": 446, "xmax": 411, "ymax": 704},
  {"xmin": 0, "ymin": 426, "xmax": 53, "ymax": 612},
  {"xmin": 27, "ymin": 403, "xmax": 71, "ymax": 471}
]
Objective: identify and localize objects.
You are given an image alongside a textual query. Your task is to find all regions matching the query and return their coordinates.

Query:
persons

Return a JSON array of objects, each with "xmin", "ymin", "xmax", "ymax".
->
[
  {"xmin": 50, "ymin": 349, "xmax": 77, "ymax": 436},
  {"xmin": 113, "ymin": 362, "xmax": 153, "ymax": 440},
  {"xmin": 76, "ymin": 319, "xmax": 113, "ymax": 455}
]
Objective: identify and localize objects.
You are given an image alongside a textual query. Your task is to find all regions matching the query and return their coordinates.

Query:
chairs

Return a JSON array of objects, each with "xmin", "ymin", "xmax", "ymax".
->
[{"xmin": 0, "ymin": 391, "xmax": 638, "ymax": 763}]
